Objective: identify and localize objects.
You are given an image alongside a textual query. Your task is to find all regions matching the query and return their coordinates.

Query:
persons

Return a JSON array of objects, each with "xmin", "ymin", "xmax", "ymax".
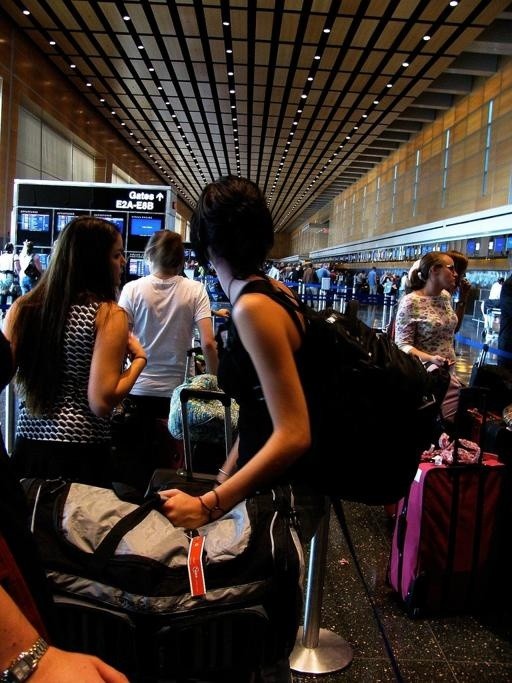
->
[{"xmin": 0, "ymin": 173, "xmax": 511, "ymax": 683}]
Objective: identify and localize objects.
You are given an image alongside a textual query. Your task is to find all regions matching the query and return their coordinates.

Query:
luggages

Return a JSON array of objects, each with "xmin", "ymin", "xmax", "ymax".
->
[
  {"xmin": 382, "ymin": 384, "xmax": 510, "ymax": 621},
  {"xmin": 166, "ymin": 339, "xmax": 238, "ymax": 443},
  {"xmin": 467, "ymin": 340, "xmax": 512, "ymax": 401},
  {"xmin": 141, "ymin": 384, "xmax": 294, "ymax": 532}
]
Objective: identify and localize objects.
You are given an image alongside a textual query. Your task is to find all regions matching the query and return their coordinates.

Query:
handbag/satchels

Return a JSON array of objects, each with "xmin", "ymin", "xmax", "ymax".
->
[
  {"xmin": 23, "ymin": 252, "xmax": 42, "ymax": 281},
  {"xmin": 0, "ymin": 474, "xmax": 302, "ymax": 682}
]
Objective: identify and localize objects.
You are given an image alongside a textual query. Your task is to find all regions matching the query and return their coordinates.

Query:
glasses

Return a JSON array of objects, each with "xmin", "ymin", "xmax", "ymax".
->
[{"xmin": 441, "ymin": 262, "xmax": 455, "ymax": 273}]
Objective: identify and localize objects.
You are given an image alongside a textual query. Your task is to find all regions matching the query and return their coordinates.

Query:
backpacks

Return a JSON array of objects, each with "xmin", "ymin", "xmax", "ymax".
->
[{"xmin": 212, "ymin": 278, "xmax": 440, "ymax": 509}]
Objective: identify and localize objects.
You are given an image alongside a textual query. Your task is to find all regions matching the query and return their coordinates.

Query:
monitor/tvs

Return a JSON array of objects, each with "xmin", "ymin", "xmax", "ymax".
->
[{"xmin": 17, "ymin": 208, "xmax": 166, "ymax": 291}]
